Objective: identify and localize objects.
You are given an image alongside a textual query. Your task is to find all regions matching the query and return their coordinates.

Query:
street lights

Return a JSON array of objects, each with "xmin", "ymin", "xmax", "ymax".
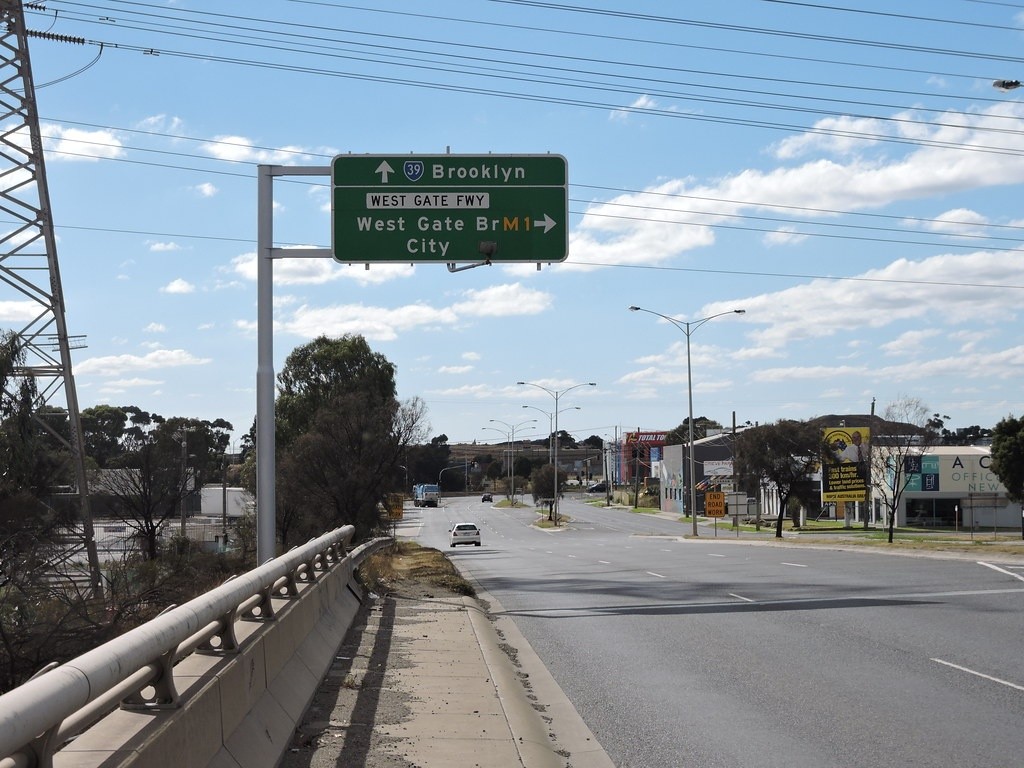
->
[
  {"xmin": 628, "ymin": 304, "xmax": 747, "ymax": 536},
  {"xmin": 522, "ymin": 405, "xmax": 581, "ymax": 467},
  {"xmin": 517, "ymin": 381, "xmax": 597, "ymax": 526},
  {"xmin": 482, "ymin": 426, "xmax": 537, "ymax": 477},
  {"xmin": 489, "ymin": 419, "xmax": 538, "ymax": 507}
]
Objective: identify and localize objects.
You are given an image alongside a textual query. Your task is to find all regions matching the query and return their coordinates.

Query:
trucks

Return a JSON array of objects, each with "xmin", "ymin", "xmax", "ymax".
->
[{"xmin": 412, "ymin": 483, "xmax": 439, "ymax": 507}]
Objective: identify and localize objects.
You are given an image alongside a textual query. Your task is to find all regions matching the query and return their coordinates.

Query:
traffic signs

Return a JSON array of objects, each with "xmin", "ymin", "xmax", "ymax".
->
[{"xmin": 329, "ymin": 152, "xmax": 569, "ymax": 264}]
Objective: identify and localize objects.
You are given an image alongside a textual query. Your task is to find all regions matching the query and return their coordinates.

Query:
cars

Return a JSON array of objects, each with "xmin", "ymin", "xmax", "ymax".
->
[
  {"xmin": 585, "ymin": 483, "xmax": 606, "ymax": 493},
  {"xmin": 482, "ymin": 493, "xmax": 493, "ymax": 503},
  {"xmin": 448, "ymin": 523, "xmax": 481, "ymax": 546}
]
